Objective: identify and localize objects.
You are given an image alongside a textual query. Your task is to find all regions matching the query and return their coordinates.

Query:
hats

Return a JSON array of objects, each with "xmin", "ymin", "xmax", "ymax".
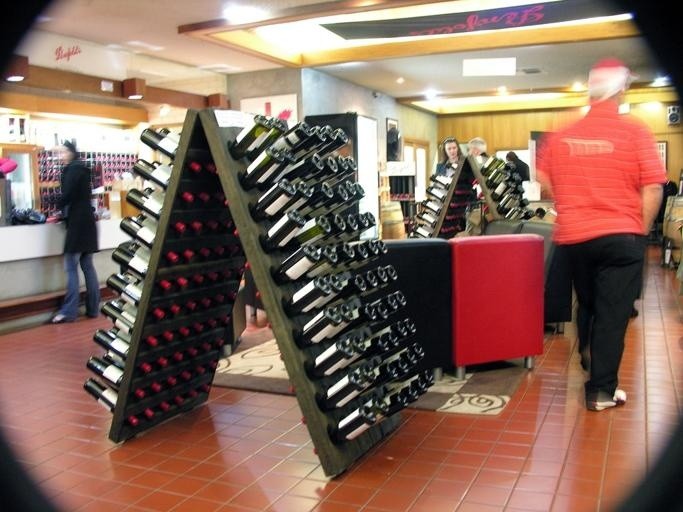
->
[{"xmin": 586, "ymin": 58, "xmax": 640, "ymax": 103}]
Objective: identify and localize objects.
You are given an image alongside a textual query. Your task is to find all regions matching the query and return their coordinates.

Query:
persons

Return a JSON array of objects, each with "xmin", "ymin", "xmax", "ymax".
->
[
  {"xmin": 42, "ymin": 139, "xmax": 104, "ymax": 326},
  {"xmin": 505, "ymin": 152, "xmax": 531, "ymax": 182},
  {"xmin": 436, "ymin": 137, "xmax": 461, "ymax": 177},
  {"xmin": 468, "ymin": 139, "xmax": 488, "ymax": 164},
  {"xmin": 535, "ymin": 58, "xmax": 668, "ymax": 412}
]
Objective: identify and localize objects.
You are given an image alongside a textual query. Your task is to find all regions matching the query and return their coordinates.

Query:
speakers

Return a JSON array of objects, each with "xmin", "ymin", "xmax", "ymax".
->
[{"xmin": 667, "ymin": 106, "xmax": 681, "ymax": 124}]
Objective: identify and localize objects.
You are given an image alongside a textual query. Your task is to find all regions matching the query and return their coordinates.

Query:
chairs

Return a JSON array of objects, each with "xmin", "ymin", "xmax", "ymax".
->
[
  {"xmin": 446, "ymin": 233, "xmax": 544, "ymax": 382},
  {"xmin": 478, "ymin": 219, "xmax": 525, "ymax": 234},
  {"xmin": 519, "ymin": 222, "xmax": 572, "ymax": 334},
  {"xmin": 343, "ymin": 237, "xmax": 453, "ymax": 380}
]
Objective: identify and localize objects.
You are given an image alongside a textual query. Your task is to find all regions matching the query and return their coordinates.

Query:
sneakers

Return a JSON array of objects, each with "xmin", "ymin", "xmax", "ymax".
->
[
  {"xmin": 52, "ymin": 312, "xmax": 66, "ymax": 324},
  {"xmin": 585, "ymin": 388, "xmax": 626, "ymax": 411}
]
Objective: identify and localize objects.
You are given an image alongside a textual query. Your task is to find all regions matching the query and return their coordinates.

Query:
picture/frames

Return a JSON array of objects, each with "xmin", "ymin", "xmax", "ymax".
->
[{"xmin": 386, "ymin": 117, "xmax": 399, "ymax": 132}]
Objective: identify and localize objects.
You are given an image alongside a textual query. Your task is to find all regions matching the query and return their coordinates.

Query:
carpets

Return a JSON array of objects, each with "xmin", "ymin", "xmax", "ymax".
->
[{"xmin": 405, "ymin": 307, "xmax": 558, "ymax": 415}]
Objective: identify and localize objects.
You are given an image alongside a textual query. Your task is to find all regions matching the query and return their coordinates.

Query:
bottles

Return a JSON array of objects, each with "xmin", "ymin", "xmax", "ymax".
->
[
  {"xmin": 678, "ymin": 169, "xmax": 683, "ymax": 196},
  {"xmin": 81, "ymin": 127, "xmax": 244, "ymax": 426},
  {"xmin": 214, "ymin": 114, "xmax": 434, "ymax": 444},
  {"xmin": 416, "ymin": 158, "xmax": 546, "ymax": 239},
  {"xmin": 663, "ymin": 239, "xmax": 671, "ymax": 267}
]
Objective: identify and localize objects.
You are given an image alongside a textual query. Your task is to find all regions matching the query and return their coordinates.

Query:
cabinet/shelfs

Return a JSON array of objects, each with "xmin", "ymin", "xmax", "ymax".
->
[{"xmin": 379, "ymin": 162, "xmax": 416, "ymax": 232}]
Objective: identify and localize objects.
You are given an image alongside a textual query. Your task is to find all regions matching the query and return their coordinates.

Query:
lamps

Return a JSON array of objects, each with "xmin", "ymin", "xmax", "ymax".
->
[
  {"xmin": 123, "ymin": 78, "xmax": 145, "ymax": 100},
  {"xmin": 207, "ymin": 94, "xmax": 229, "ymax": 110},
  {"xmin": 4, "ymin": 55, "xmax": 29, "ymax": 82}
]
